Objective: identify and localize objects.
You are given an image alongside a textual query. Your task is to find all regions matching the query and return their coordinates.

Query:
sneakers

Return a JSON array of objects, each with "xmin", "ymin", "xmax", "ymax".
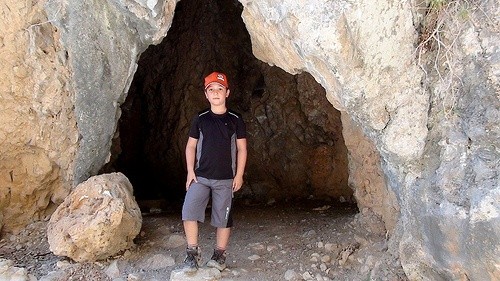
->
[
  {"xmin": 206, "ymin": 249, "xmax": 227, "ymax": 272},
  {"xmin": 182, "ymin": 245, "xmax": 203, "ymax": 273}
]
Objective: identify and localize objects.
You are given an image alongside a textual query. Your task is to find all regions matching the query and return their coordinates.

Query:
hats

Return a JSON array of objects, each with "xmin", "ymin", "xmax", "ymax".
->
[{"xmin": 205, "ymin": 71, "xmax": 228, "ymax": 91}]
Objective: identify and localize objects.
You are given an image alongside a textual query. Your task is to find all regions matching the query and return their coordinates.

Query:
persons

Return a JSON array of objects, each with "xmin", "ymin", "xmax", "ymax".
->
[{"xmin": 181, "ymin": 71, "xmax": 247, "ymax": 273}]
